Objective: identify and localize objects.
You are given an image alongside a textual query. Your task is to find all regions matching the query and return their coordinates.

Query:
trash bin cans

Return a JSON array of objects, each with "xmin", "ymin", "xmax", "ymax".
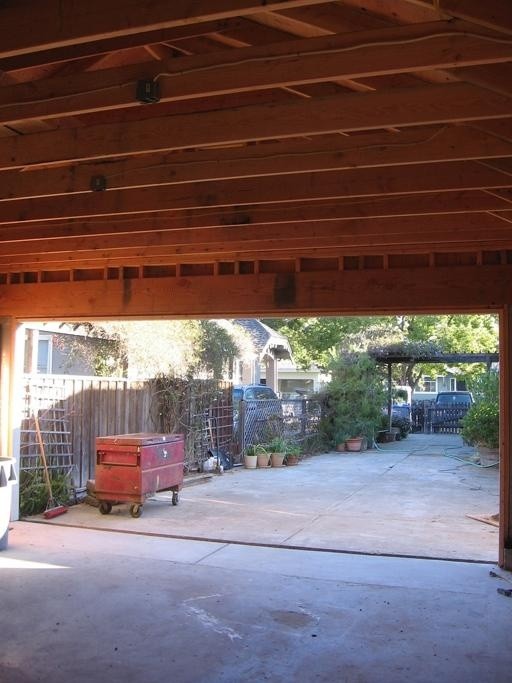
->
[{"xmin": 0, "ymin": 456, "xmax": 16, "ymax": 550}]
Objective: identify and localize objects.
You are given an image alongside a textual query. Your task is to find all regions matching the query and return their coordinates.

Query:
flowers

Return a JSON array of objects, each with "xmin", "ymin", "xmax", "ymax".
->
[{"xmin": 459, "ymin": 397, "xmax": 499, "ymax": 449}]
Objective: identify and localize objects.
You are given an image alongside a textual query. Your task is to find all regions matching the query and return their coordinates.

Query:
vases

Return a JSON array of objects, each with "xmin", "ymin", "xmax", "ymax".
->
[{"xmin": 475, "ymin": 443, "xmax": 499, "ymax": 468}]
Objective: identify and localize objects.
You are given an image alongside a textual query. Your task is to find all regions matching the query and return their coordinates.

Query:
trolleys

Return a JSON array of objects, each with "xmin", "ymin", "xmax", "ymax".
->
[{"xmin": 90, "ymin": 428, "xmax": 188, "ymax": 516}]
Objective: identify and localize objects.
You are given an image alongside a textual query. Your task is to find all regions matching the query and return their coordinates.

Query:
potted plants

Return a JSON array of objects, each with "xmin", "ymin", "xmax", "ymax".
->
[
  {"xmin": 243, "ymin": 437, "xmax": 305, "ymax": 468},
  {"xmin": 332, "ymin": 423, "xmax": 365, "ymax": 451}
]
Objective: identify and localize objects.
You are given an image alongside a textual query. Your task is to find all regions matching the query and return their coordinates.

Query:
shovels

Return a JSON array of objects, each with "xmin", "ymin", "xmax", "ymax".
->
[{"xmin": 208, "ymin": 418, "xmax": 229, "ymax": 470}]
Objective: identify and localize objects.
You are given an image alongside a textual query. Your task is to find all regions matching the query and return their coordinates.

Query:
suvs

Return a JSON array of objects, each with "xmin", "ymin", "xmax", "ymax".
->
[
  {"xmin": 435, "ymin": 390, "xmax": 475, "ymax": 433},
  {"xmin": 234, "ymin": 383, "xmax": 285, "ymax": 447}
]
